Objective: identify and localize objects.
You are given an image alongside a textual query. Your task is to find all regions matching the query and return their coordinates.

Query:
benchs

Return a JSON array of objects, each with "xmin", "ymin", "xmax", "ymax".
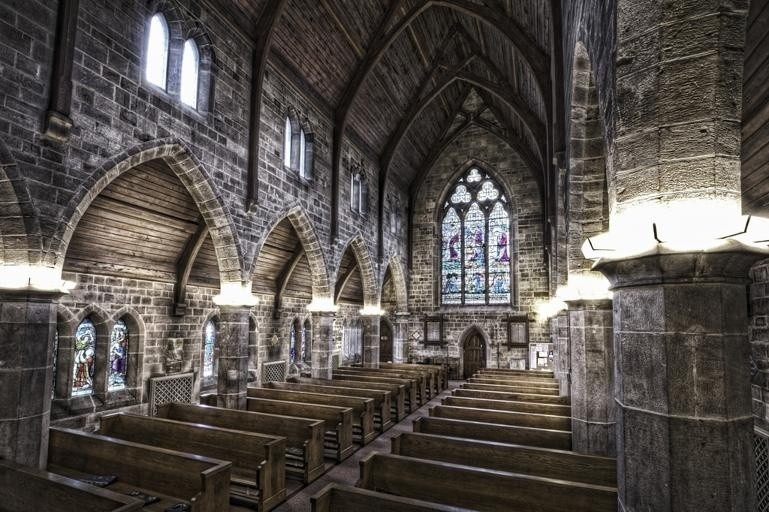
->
[
  {"xmin": 0, "ymin": 363, "xmax": 461, "ymax": 512},
  {"xmin": 309, "ymin": 368, "xmax": 621, "ymax": 511}
]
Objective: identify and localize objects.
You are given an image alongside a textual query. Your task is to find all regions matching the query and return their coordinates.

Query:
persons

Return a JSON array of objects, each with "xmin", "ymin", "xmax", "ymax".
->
[
  {"xmin": 72, "ymin": 330, "xmax": 94, "ymax": 391},
  {"xmin": 110, "ymin": 327, "xmax": 128, "ymax": 388}
]
